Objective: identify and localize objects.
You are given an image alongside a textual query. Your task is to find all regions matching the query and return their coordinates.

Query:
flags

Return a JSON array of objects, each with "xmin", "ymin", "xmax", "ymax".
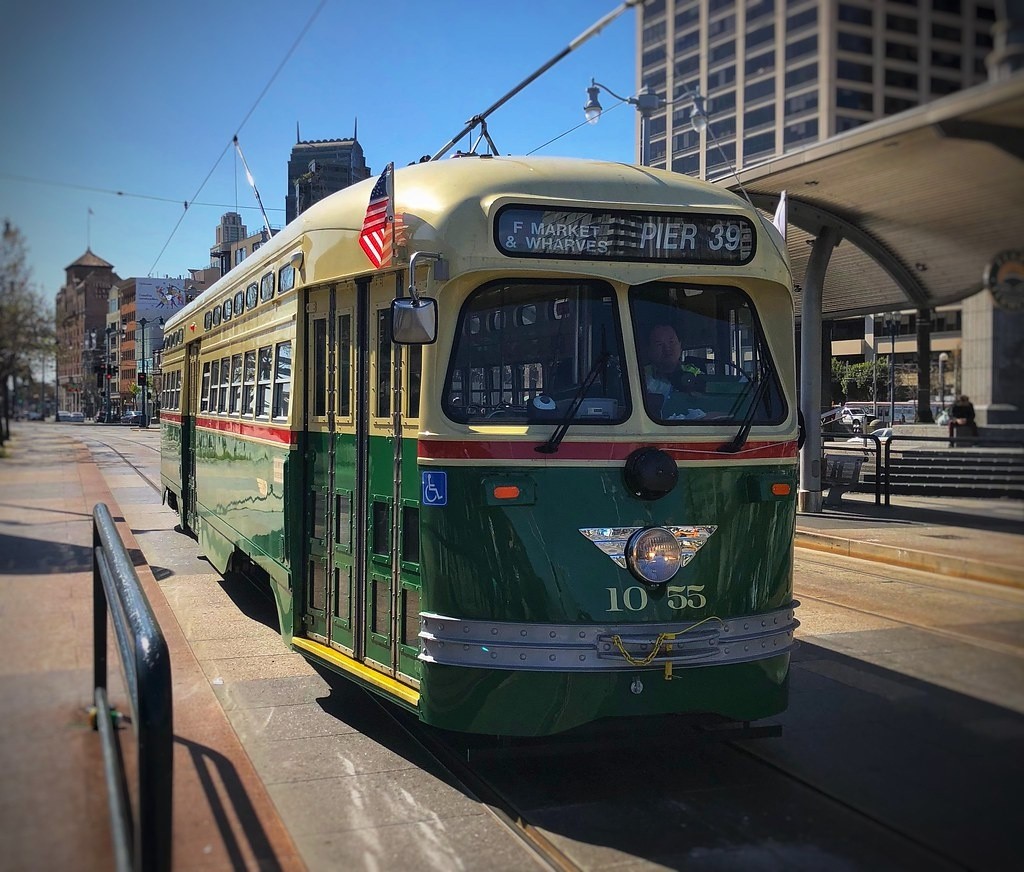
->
[
  {"xmin": 358, "ymin": 164, "xmax": 393, "ymax": 269},
  {"xmin": 774, "ymin": 195, "xmax": 787, "ymax": 240}
]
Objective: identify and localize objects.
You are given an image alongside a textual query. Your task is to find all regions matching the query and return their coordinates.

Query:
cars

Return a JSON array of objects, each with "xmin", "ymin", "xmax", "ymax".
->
[
  {"xmin": 71, "ymin": 412, "xmax": 85, "ymax": 423},
  {"xmin": 59, "ymin": 411, "xmax": 72, "ymax": 421},
  {"xmin": 93, "ymin": 411, "xmax": 105, "ymax": 423},
  {"xmin": 120, "ymin": 410, "xmax": 143, "ymax": 424},
  {"xmin": 842, "ymin": 408, "xmax": 869, "ymax": 427},
  {"xmin": 24, "ymin": 410, "xmax": 43, "ymax": 420}
]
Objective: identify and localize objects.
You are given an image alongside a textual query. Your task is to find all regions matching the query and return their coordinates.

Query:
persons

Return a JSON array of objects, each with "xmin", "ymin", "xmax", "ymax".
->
[
  {"xmin": 643, "ymin": 324, "xmax": 704, "ymax": 412},
  {"xmin": 947, "ymin": 395, "xmax": 979, "ymax": 448}
]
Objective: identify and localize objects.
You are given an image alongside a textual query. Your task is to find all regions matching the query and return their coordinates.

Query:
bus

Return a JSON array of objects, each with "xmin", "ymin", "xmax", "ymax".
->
[
  {"xmin": 152, "ymin": 112, "xmax": 805, "ymax": 740},
  {"xmin": 845, "ymin": 402, "xmax": 938, "ymax": 423}
]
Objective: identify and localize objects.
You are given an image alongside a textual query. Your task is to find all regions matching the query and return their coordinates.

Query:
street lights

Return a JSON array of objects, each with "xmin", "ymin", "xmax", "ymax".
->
[
  {"xmin": 165, "ymin": 282, "xmax": 205, "ymax": 303},
  {"xmin": 938, "ymin": 352, "xmax": 948, "ymax": 412},
  {"xmin": 122, "ymin": 315, "xmax": 165, "ymax": 425},
  {"xmin": 91, "ymin": 324, "xmax": 126, "ymax": 420},
  {"xmin": 582, "ymin": 77, "xmax": 714, "ymax": 168}
]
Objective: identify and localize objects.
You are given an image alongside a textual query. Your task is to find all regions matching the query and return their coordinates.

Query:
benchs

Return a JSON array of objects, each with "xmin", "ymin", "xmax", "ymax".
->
[{"xmin": 821, "ymin": 453, "xmax": 869, "ymax": 506}]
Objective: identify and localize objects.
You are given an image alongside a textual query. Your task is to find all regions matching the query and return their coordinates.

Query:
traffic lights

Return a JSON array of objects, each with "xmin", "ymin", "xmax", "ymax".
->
[
  {"xmin": 106, "ymin": 363, "xmax": 112, "ymax": 379},
  {"xmin": 100, "ymin": 364, "xmax": 105, "ymax": 374},
  {"xmin": 138, "ymin": 373, "xmax": 146, "ymax": 385},
  {"xmin": 114, "ymin": 366, "xmax": 118, "ymax": 375}
]
